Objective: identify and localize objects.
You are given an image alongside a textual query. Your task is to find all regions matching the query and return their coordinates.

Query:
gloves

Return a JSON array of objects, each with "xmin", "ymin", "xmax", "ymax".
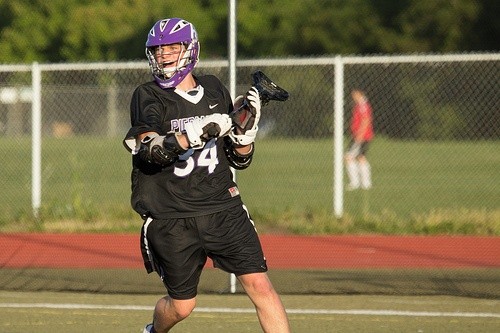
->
[
  {"xmin": 185, "ymin": 113, "xmax": 233, "ymax": 151},
  {"xmin": 228, "ymin": 87, "xmax": 263, "ymax": 146}
]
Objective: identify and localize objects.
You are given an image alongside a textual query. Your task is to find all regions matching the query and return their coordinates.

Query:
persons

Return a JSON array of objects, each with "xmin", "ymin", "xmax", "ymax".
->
[
  {"xmin": 344, "ymin": 83, "xmax": 375, "ymax": 190},
  {"xmin": 121, "ymin": 17, "xmax": 296, "ymax": 333}
]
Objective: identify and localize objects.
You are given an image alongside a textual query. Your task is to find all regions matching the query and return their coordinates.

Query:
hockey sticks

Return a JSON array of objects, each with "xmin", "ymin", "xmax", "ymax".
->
[{"xmin": 207, "ymin": 71, "xmax": 289, "ymax": 140}]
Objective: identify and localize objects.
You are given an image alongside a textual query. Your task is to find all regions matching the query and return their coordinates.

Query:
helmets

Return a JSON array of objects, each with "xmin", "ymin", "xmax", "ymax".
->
[{"xmin": 146, "ymin": 17, "xmax": 200, "ymax": 89}]
